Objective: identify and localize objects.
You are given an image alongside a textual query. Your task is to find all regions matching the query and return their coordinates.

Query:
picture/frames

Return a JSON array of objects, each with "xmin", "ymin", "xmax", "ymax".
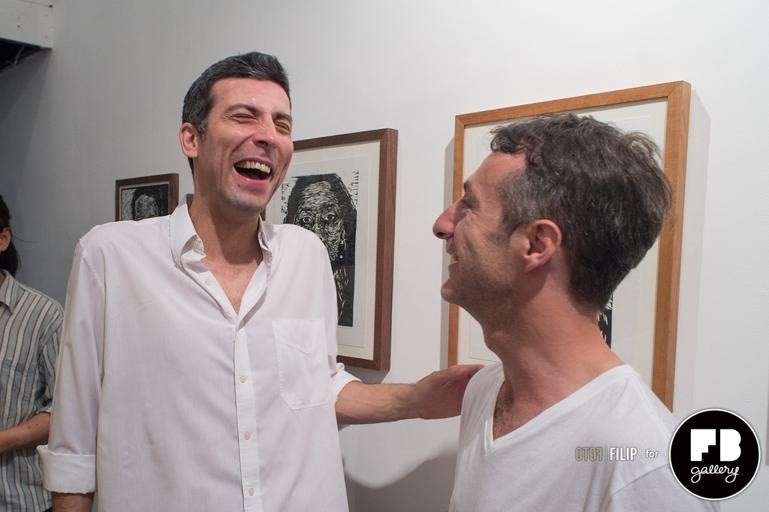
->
[
  {"xmin": 444, "ymin": 78, "xmax": 693, "ymax": 413},
  {"xmin": 258, "ymin": 128, "xmax": 399, "ymax": 373},
  {"xmin": 115, "ymin": 172, "xmax": 179, "ymax": 221}
]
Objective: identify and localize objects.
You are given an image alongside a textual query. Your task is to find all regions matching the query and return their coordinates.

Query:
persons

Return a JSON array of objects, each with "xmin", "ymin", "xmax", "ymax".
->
[
  {"xmin": 0, "ymin": 197, "xmax": 65, "ymax": 512},
  {"xmin": 131, "ymin": 188, "xmax": 162, "ymax": 221},
  {"xmin": 37, "ymin": 49, "xmax": 484, "ymax": 512},
  {"xmin": 283, "ymin": 172, "xmax": 356, "ymax": 327},
  {"xmin": 431, "ymin": 112, "xmax": 720, "ymax": 512}
]
